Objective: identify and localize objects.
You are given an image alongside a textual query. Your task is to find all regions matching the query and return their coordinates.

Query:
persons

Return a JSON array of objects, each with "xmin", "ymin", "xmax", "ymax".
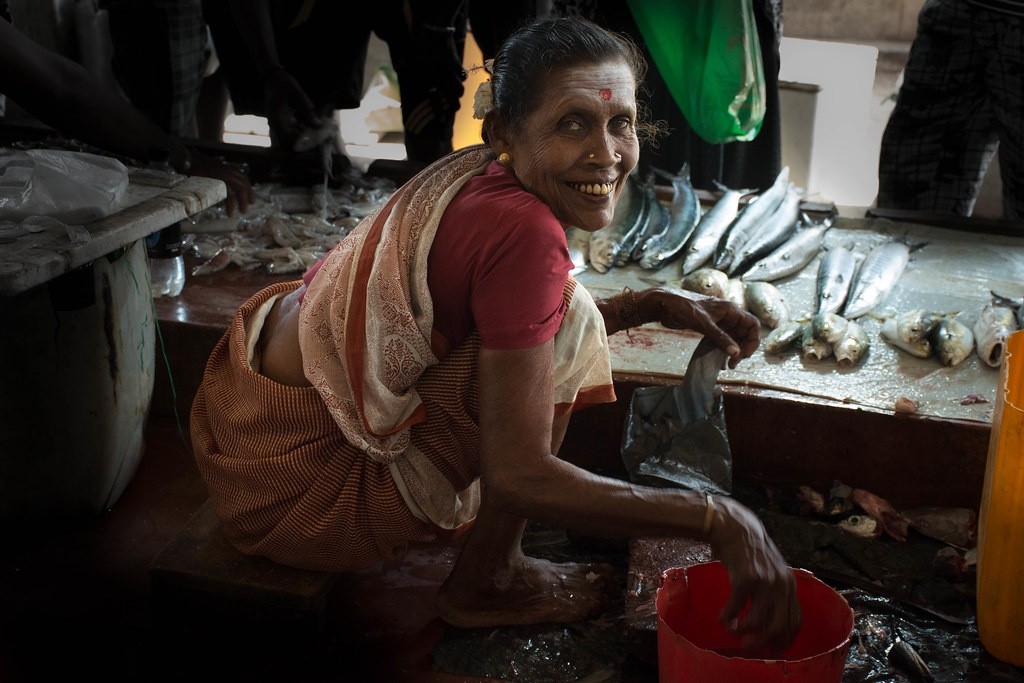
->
[
  {"xmin": 0, "ymin": 0, "xmax": 781, "ymax": 288},
  {"xmin": 876, "ymin": 0, "xmax": 1024, "ymax": 228},
  {"xmin": 187, "ymin": 16, "xmax": 802, "ymax": 657}
]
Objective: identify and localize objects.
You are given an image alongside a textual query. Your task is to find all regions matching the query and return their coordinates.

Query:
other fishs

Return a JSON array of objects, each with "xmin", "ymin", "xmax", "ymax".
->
[
  {"xmin": 772, "ymin": 476, "xmax": 977, "ymax": 683},
  {"xmin": 563, "ymin": 158, "xmax": 1023, "ymax": 369},
  {"xmin": 169, "ymin": 182, "xmax": 391, "ymax": 277}
]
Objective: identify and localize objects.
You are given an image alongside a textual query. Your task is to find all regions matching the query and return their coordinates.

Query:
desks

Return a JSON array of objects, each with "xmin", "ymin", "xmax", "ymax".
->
[
  {"xmin": 1, "ymin": 148, "xmax": 229, "ymax": 514},
  {"xmin": 149, "ymin": 180, "xmax": 1024, "ymax": 546}
]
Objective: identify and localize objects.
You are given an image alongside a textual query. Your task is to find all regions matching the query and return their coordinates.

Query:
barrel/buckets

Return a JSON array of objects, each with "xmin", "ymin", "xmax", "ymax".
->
[
  {"xmin": 977, "ymin": 330, "xmax": 1024, "ymax": 667},
  {"xmin": 654, "ymin": 561, "xmax": 854, "ymax": 683}
]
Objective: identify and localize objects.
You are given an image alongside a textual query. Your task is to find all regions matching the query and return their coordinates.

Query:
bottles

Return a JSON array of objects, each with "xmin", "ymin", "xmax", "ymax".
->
[{"xmin": 146, "ymin": 160, "xmax": 185, "ymax": 297}]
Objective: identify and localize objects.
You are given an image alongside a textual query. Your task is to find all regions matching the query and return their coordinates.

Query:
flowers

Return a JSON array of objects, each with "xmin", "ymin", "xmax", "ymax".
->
[{"xmin": 461, "ymin": 58, "xmax": 493, "ymax": 119}]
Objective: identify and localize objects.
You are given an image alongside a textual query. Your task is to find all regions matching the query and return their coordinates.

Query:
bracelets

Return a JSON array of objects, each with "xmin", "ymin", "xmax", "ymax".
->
[
  {"xmin": 607, "ymin": 286, "xmax": 642, "ymax": 332},
  {"xmin": 701, "ymin": 491, "xmax": 714, "ymax": 540}
]
